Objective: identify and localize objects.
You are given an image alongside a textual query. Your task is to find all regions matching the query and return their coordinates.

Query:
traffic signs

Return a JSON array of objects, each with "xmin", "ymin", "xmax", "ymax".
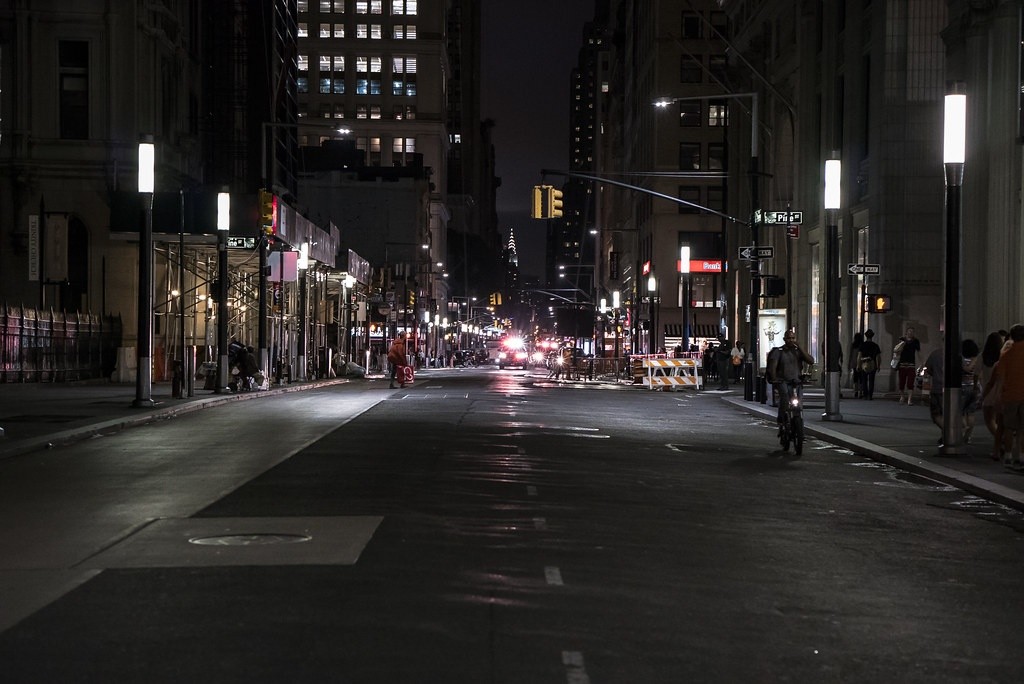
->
[
  {"xmin": 848, "ymin": 263, "xmax": 882, "ymax": 275},
  {"xmin": 737, "ymin": 246, "xmax": 774, "ymax": 260}
]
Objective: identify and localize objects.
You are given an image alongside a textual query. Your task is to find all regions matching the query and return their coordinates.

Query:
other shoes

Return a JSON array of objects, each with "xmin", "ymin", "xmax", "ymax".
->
[
  {"xmin": 717, "ymin": 385, "xmax": 728, "ymax": 390},
  {"xmin": 963, "ymin": 425, "xmax": 974, "ymax": 442},
  {"xmin": 989, "ymin": 451, "xmax": 1024, "ymax": 470},
  {"xmin": 390, "ymin": 387, "xmax": 397, "ymax": 389},
  {"xmin": 401, "ymin": 386, "xmax": 408, "ymax": 388}
]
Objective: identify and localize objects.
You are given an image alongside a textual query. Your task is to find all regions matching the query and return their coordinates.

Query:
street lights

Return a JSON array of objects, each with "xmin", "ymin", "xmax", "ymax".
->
[
  {"xmin": 215, "ymin": 183, "xmax": 233, "ymax": 394},
  {"xmin": 590, "ymin": 226, "xmax": 640, "ymax": 353},
  {"xmin": 943, "ymin": 77, "xmax": 970, "ymax": 460},
  {"xmin": 381, "ymin": 240, "xmax": 430, "ymax": 374},
  {"xmin": 295, "ymin": 241, "xmax": 311, "ymax": 382},
  {"xmin": 559, "ymin": 264, "xmax": 599, "ymax": 308},
  {"xmin": 450, "ymin": 291, "xmax": 478, "ymax": 368},
  {"xmin": 679, "ymin": 242, "xmax": 691, "ymax": 352},
  {"xmin": 653, "ymin": 90, "xmax": 763, "ymax": 402},
  {"xmin": 132, "ymin": 132, "xmax": 159, "ymax": 407},
  {"xmin": 821, "ymin": 149, "xmax": 845, "ymax": 418},
  {"xmin": 424, "ymin": 311, "xmax": 448, "ymax": 369},
  {"xmin": 340, "ymin": 275, "xmax": 358, "ymax": 363},
  {"xmin": 599, "ymin": 289, "xmax": 621, "ymax": 362},
  {"xmin": 403, "ymin": 243, "xmax": 449, "ymax": 353}
]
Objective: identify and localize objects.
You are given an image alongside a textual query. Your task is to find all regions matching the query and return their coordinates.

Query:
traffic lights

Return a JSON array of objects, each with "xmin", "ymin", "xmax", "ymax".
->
[
  {"xmin": 864, "ymin": 293, "xmax": 892, "ymax": 313},
  {"xmin": 490, "ymin": 293, "xmax": 496, "ymax": 305},
  {"xmin": 547, "ymin": 189, "xmax": 564, "ymax": 219},
  {"xmin": 259, "ymin": 189, "xmax": 277, "ymax": 233},
  {"xmin": 408, "ymin": 290, "xmax": 415, "ymax": 304}
]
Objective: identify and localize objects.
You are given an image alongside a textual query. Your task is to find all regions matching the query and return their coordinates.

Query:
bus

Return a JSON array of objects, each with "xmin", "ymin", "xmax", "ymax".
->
[{"xmin": 486, "ymin": 339, "xmax": 503, "ymax": 364}]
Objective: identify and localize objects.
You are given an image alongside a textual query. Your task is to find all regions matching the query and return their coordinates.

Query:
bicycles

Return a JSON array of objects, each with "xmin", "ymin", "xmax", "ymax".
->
[{"xmin": 770, "ymin": 378, "xmax": 819, "ymax": 456}]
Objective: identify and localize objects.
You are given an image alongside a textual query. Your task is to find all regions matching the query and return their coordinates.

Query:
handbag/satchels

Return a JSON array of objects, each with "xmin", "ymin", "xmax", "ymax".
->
[
  {"xmin": 397, "ymin": 364, "xmax": 414, "ymax": 383},
  {"xmin": 891, "ymin": 353, "xmax": 901, "ymax": 368},
  {"xmin": 733, "ymin": 357, "xmax": 741, "ymax": 365}
]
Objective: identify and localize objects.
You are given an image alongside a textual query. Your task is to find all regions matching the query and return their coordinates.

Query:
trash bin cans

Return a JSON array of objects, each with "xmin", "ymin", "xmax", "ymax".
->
[
  {"xmin": 202, "ymin": 362, "xmax": 217, "ymax": 391},
  {"xmin": 377, "ymin": 354, "xmax": 387, "ymax": 371},
  {"xmin": 319, "ymin": 346, "xmax": 332, "ymax": 378},
  {"xmin": 172, "ymin": 360, "xmax": 189, "ymax": 397}
]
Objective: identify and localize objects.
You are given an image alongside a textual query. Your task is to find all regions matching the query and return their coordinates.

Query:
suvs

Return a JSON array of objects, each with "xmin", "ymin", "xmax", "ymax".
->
[
  {"xmin": 453, "ymin": 347, "xmax": 489, "ymax": 368},
  {"xmin": 546, "ymin": 347, "xmax": 593, "ymax": 380}
]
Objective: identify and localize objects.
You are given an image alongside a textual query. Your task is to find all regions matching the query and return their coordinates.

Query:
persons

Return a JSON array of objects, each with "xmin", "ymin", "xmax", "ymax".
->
[
  {"xmin": 246, "ymin": 345, "xmax": 264, "ymax": 386},
  {"xmin": 849, "ymin": 329, "xmax": 882, "ymax": 402},
  {"xmin": 769, "ymin": 330, "xmax": 813, "ymax": 444},
  {"xmin": 923, "ymin": 323, "xmax": 1024, "ymax": 469},
  {"xmin": 388, "ymin": 331, "xmax": 408, "ymax": 389},
  {"xmin": 893, "ymin": 327, "xmax": 923, "ymax": 406},
  {"xmin": 703, "ymin": 332, "xmax": 747, "ymax": 391},
  {"xmin": 623, "ymin": 347, "xmax": 644, "ymax": 379},
  {"xmin": 372, "ymin": 346, "xmax": 384, "ymax": 370}
]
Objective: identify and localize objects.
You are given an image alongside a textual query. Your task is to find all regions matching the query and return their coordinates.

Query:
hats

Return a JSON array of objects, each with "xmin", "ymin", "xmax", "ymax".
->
[{"xmin": 865, "ymin": 329, "xmax": 875, "ymax": 337}]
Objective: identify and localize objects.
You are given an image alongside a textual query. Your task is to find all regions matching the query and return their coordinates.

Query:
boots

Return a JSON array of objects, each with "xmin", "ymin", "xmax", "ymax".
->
[
  {"xmin": 907, "ymin": 390, "xmax": 914, "ymax": 405},
  {"xmin": 899, "ymin": 389, "xmax": 905, "ymax": 402}
]
